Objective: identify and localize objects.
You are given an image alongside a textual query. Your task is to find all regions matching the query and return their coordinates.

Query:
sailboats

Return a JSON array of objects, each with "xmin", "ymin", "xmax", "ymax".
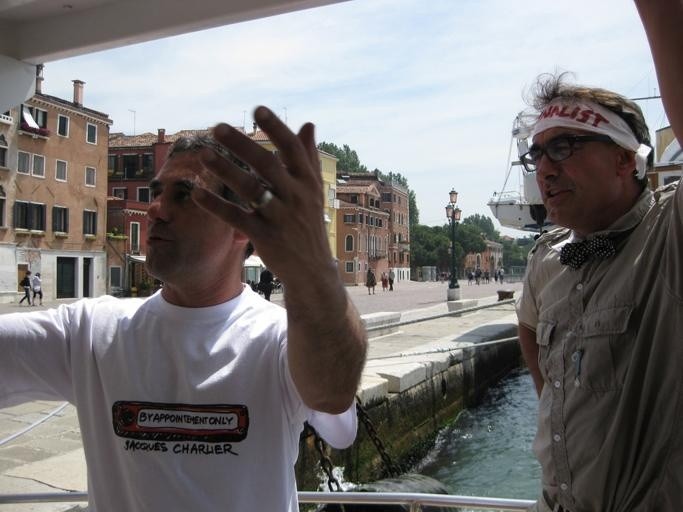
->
[{"xmin": 487, "ymin": 110, "xmax": 557, "ymax": 235}]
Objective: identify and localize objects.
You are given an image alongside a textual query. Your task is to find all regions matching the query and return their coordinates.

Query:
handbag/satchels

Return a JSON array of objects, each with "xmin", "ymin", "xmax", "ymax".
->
[
  {"xmin": 257, "ymin": 282, "xmax": 274, "ymax": 292},
  {"xmin": 20, "ymin": 280, "xmax": 24, "ymax": 287},
  {"xmin": 367, "ymin": 279, "xmax": 376, "ymax": 287}
]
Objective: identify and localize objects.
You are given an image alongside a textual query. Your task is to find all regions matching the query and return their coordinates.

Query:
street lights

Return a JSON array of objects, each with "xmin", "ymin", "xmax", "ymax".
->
[{"xmin": 444, "ymin": 188, "xmax": 462, "ymax": 288}]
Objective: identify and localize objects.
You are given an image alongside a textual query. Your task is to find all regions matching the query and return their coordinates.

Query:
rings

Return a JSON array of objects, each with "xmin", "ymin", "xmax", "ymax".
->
[{"xmin": 250, "ymin": 189, "xmax": 274, "ymax": 209}]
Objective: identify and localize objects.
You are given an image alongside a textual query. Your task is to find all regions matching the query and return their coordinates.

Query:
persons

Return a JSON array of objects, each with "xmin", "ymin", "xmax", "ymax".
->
[
  {"xmin": 513, "ymin": 1, "xmax": 683, "ymax": 512},
  {"xmin": 32, "ymin": 272, "xmax": 43, "ymax": 306},
  {"xmin": 465, "ymin": 265, "xmax": 505, "ymax": 283},
  {"xmin": 19, "ymin": 271, "xmax": 32, "ymax": 305},
  {"xmin": 366, "ymin": 267, "xmax": 395, "ymax": 295},
  {"xmin": 259, "ymin": 267, "xmax": 273, "ymax": 302},
  {"xmin": 0, "ymin": 103, "xmax": 368, "ymax": 512}
]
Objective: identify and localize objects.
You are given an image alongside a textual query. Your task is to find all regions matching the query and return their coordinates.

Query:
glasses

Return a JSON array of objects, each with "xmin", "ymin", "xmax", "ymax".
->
[{"xmin": 521, "ymin": 131, "xmax": 612, "ymax": 172}]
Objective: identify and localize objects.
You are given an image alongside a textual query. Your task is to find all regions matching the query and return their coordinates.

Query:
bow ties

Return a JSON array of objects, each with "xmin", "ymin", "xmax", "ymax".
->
[{"xmin": 560, "ymin": 231, "xmax": 617, "ymax": 269}]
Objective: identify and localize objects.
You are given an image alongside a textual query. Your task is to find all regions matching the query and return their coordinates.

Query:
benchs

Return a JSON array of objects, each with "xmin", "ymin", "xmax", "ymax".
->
[
  {"xmin": 360, "ymin": 311, "xmax": 401, "ymax": 338},
  {"xmin": 447, "ymin": 299, "xmax": 479, "ymax": 317},
  {"xmin": 496, "ymin": 290, "xmax": 515, "ymax": 301}
]
